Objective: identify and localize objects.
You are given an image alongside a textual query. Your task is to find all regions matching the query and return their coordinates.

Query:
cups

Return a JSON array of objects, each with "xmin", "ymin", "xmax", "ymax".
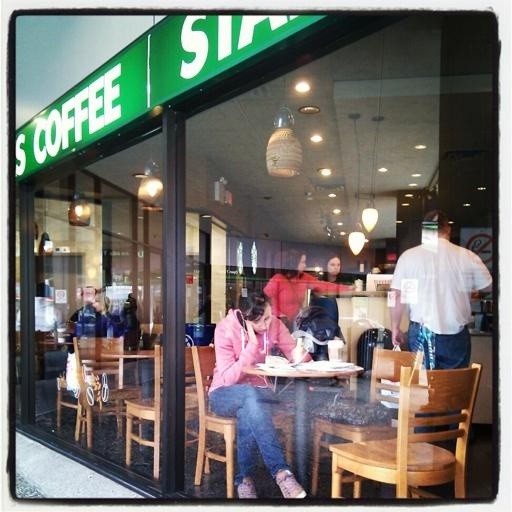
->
[
  {"xmin": 327, "ymin": 339, "xmax": 344, "ymax": 362},
  {"xmin": 355, "ymin": 279, "xmax": 363, "ymax": 291}
]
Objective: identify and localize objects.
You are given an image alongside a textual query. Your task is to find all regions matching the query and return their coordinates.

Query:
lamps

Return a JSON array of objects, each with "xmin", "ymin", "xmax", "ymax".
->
[
  {"xmin": 348, "ymin": 113, "xmax": 385, "ymax": 256},
  {"xmin": 264, "ymin": 75, "xmax": 305, "ymax": 179},
  {"xmin": 69, "ymin": 196, "xmax": 91, "ymax": 227},
  {"xmin": 137, "ymin": 174, "xmax": 164, "ymax": 204}
]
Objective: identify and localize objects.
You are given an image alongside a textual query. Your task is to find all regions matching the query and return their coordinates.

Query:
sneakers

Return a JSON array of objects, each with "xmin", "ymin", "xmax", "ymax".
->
[
  {"xmin": 274, "ymin": 470, "xmax": 308, "ymax": 499},
  {"xmin": 236, "ymin": 479, "xmax": 259, "ymax": 499}
]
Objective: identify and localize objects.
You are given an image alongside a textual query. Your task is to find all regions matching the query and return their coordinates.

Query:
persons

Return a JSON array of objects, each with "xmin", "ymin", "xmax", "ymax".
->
[
  {"xmin": 205, "ymin": 287, "xmax": 314, "ymax": 501},
  {"xmin": 389, "ymin": 210, "xmax": 494, "ymax": 500},
  {"xmin": 15, "ymin": 283, "xmax": 143, "ymax": 407},
  {"xmin": 311, "ymin": 251, "xmax": 355, "ymax": 319},
  {"xmin": 262, "ymin": 247, "xmax": 360, "ymax": 334}
]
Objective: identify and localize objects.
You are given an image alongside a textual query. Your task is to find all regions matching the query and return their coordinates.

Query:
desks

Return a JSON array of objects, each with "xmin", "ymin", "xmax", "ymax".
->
[
  {"xmin": 242, "ymin": 359, "xmax": 365, "ymax": 499},
  {"xmin": 100, "ymin": 348, "xmax": 153, "ymax": 451}
]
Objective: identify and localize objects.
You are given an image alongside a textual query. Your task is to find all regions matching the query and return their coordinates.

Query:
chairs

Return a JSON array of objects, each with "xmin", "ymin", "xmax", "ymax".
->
[
  {"xmin": 122, "ymin": 345, "xmax": 196, "ymax": 480},
  {"xmin": 310, "ymin": 348, "xmax": 424, "ymax": 496},
  {"xmin": 191, "ymin": 343, "xmax": 294, "ymax": 498},
  {"xmin": 329, "ymin": 360, "xmax": 484, "ymax": 498},
  {"xmin": 58, "ymin": 334, "xmax": 143, "ymax": 450}
]
